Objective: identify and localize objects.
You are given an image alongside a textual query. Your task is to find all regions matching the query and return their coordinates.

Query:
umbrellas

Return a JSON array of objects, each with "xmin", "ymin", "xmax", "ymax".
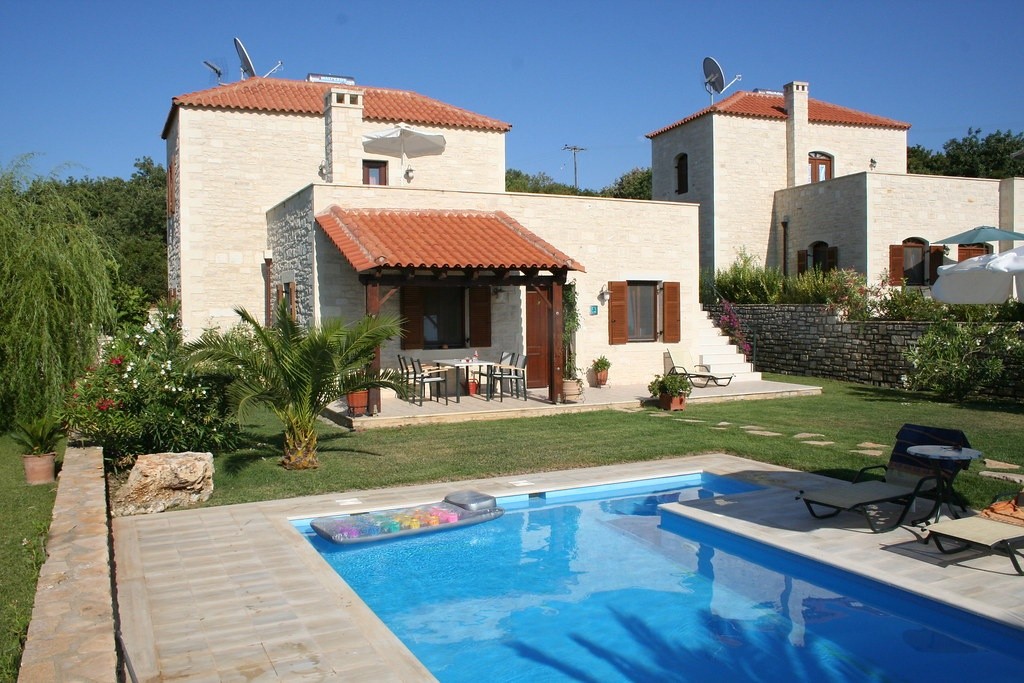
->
[{"xmin": 929, "ymin": 226, "xmax": 1024, "ymax": 255}]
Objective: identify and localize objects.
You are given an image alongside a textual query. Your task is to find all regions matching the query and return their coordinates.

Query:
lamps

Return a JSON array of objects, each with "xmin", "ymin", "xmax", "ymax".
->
[
  {"xmin": 871, "ymin": 158, "xmax": 877, "ymax": 168},
  {"xmin": 494, "ymin": 286, "xmax": 505, "ymax": 301},
  {"xmin": 405, "ymin": 164, "xmax": 415, "ymax": 179},
  {"xmin": 599, "ymin": 284, "xmax": 614, "ymax": 300},
  {"xmin": 319, "ymin": 160, "xmax": 328, "ymax": 175},
  {"xmin": 943, "ymin": 245, "xmax": 950, "ymax": 256}
]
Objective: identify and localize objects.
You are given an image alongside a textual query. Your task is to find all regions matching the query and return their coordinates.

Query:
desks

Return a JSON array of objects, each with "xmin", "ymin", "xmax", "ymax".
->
[
  {"xmin": 907, "ymin": 445, "xmax": 983, "ymax": 546},
  {"xmin": 433, "ymin": 360, "xmax": 496, "ymax": 405}
]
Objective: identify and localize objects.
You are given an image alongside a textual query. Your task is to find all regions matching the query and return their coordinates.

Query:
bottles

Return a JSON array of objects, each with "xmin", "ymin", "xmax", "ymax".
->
[{"xmin": 474, "ymin": 351, "xmax": 478, "ymax": 362}]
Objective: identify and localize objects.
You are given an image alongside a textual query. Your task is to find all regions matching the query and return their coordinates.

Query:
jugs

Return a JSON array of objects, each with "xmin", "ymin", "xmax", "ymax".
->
[{"xmin": 460, "ymin": 379, "xmax": 478, "ymax": 394}]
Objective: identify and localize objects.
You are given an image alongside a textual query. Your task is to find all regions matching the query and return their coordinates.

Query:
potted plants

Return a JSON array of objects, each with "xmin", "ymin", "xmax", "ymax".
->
[
  {"xmin": 592, "ymin": 355, "xmax": 611, "ymax": 386},
  {"xmin": 9, "ymin": 402, "xmax": 68, "ymax": 485},
  {"xmin": 560, "ymin": 282, "xmax": 586, "ymax": 403},
  {"xmin": 648, "ymin": 372, "xmax": 692, "ymax": 411},
  {"xmin": 344, "ymin": 371, "xmax": 368, "ymax": 417}
]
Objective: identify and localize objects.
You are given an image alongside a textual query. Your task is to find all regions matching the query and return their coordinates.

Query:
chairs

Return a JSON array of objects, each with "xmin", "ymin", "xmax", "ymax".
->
[
  {"xmin": 397, "ymin": 354, "xmax": 433, "ymax": 400},
  {"xmin": 478, "ymin": 351, "xmax": 516, "ymax": 398},
  {"xmin": 410, "ymin": 357, "xmax": 448, "ymax": 407},
  {"xmin": 795, "ymin": 422, "xmax": 973, "ymax": 534},
  {"xmin": 666, "ymin": 348, "xmax": 736, "ymax": 389},
  {"xmin": 492, "ymin": 353, "xmax": 528, "ymax": 402},
  {"xmin": 921, "ymin": 492, "xmax": 1024, "ymax": 576}
]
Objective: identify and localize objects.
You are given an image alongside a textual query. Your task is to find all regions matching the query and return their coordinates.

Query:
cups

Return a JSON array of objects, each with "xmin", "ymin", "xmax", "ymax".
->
[{"xmin": 465, "ymin": 356, "xmax": 470, "ymax": 363}]
